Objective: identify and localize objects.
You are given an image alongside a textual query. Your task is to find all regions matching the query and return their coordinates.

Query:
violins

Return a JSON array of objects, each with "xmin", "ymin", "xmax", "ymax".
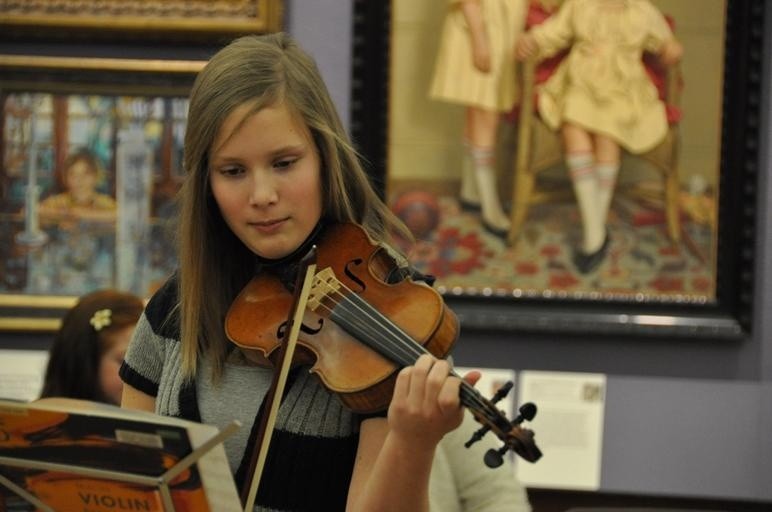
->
[{"xmin": 225, "ymin": 221, "xmax": 543, "ymax": 468}]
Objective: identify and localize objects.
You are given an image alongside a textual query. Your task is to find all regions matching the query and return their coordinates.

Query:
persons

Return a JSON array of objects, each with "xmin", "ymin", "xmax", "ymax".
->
[
  {"xmin": 515, "ymin": 1, "xmax": 687, "ymax": 276},
  {"xmin": 426, "ymin": 395, "xmax": 535, "ymax": 511},
  {"xmin": 426, "ymin": 0, "xmax": 522, "ymax": 235},
  {"xmin": 115, "ymin": 27, "xmax": 483, "ymax": 511},
  {"xmin": 39, "ymin": 286, "xmax": 141, "ymax": 412},
  {"xmin": 22, "ymin": 142, "xmax": 116, "ymax": 225}
]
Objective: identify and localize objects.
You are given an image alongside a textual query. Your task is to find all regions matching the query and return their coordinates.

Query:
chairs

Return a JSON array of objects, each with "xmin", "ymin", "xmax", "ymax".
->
[{"xmin": 502, "ymin": 2, "xmax": 688, "ymax": 249}]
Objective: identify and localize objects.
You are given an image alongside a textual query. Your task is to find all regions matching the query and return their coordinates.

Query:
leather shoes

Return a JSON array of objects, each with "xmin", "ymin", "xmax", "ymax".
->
[
  {"xmin": 575, "ymin": 232, "xmax": 612, "ymax": 274},
  {"xmin": 483, "ymin": 215, "xmax": 509, "ymax": 237},
  {"xmin": 459, "ymin": 197, "xmax": 483, "ymax": 212}
]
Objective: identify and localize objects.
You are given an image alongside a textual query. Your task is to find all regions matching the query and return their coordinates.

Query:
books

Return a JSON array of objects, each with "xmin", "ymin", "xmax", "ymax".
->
[{"xmin": 453, "ymin": 364, "xmax": 612, "ymax": 495}]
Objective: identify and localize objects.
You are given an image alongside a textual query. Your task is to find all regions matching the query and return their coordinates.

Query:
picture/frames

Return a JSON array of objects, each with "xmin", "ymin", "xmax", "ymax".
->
[
  {"xmin": 347, "ymin": 1, "xmax": 769, "ymax": 346},
  {"xmin": 1, "ymin": 0, "xmax": 285, "ymax": 42},
  {"xmin": 2, "ymin": 37, "xmax": 226, "ymax": 349}
]
